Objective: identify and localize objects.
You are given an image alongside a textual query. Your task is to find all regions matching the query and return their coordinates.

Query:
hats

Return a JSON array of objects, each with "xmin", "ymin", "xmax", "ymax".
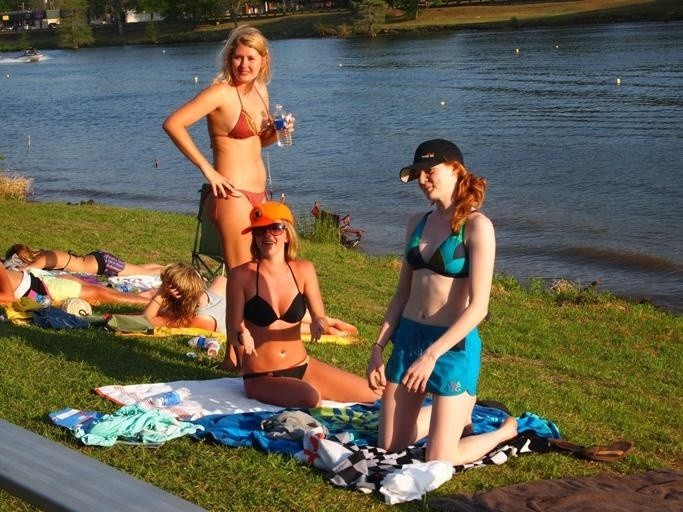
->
[
  {"xmin": 240, "ymin": 202, "xmax": 296, "ymax": 235},
  {"xmin": 400, "ymin": 139, "xmax": 465, "ymax": 184}
]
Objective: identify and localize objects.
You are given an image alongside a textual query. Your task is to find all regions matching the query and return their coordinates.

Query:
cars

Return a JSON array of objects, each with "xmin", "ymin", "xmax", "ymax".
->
[{"xmin": 88, "ymin": 19, "xmax": 105, "ymax": 25}]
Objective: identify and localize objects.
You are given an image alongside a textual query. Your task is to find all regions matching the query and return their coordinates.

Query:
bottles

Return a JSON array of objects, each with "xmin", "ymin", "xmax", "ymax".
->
[
  {"xmin": 115, "ymin": 284, "xmax": 135, "ymax": 294},
  {"xmin": 154, "ymin": 387, "xmax": 191, "ymax": 408},
  {"xmin": 271, "ymin": 102, "xmax": 292, "ymax": 148},
  {"xmin": 186, "ymin": 336, "xmax": 220, "ymax": 357}
]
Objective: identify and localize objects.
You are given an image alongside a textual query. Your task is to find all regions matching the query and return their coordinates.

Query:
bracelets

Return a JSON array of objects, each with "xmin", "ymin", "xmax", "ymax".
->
[
  {"xmin": 237, "ymin": 331, "xmax": 243, "ymax": 345},
  {"xmin": 374, "ymin": 343, "xmax": 384, "ymax": 351},
  {"xmin": 152, "ymin": 297, "xmax": 162, "ymax": 305}
]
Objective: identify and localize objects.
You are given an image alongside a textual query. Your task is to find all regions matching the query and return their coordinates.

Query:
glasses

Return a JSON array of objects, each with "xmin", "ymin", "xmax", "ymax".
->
[{"xmin": 250, "ymin": 224, "xmax": 285, "ymax": 236}]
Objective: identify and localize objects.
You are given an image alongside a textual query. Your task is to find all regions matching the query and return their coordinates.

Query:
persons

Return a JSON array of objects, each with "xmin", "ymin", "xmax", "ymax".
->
[
  {"xmin": 0, "ymin": 264, "xmax": 14, "ymax": 303},
  {"xmin": 162, "ymin": 25, "xmax": 296, "ymax": 370},
  {"xmin": 5, "ymin": 269, "xmax": 157, "ymax": 305},
  {"xmin": 143, "ymin": 262, "xmax": 227, "ymax": 333},
  {"xmin": 5, "ymin": 244, "xmax": 164, "ymax": 277},
  {"xmin": 367, "ymin": 138, "xmax": 518, "ymax": 466},
  {"xmin": 226, "ymin": 201, "xmax": 382, "ymax": 407}
]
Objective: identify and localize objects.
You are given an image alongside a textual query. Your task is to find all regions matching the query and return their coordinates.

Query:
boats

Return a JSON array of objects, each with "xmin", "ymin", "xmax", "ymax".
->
[{"xmin": 15, "ymin": 52, "xmax": 43, "ymax": 60}]
